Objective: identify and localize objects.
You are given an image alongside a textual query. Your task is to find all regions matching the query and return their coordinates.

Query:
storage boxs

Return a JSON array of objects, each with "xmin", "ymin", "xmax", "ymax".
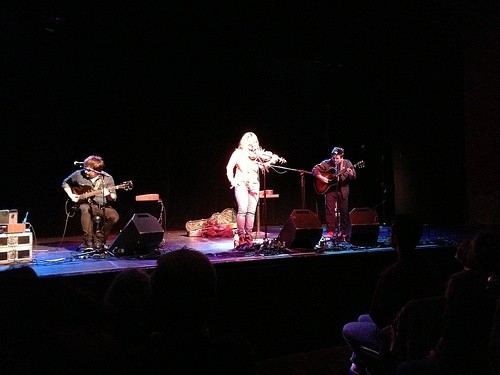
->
[{"xmin": 0, "ymin": 232, "xmax": 33, "ymax": 265}]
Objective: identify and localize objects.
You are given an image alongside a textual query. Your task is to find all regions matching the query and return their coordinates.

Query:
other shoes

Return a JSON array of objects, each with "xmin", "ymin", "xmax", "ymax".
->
[
  {"xmin": 238, "ymin": 236, "xmax": 253, "ymax": 245},
  {"xmin": 93, "ymin": 241, "xmax": 109, "ymax": 249},
  {"xmin": 328, "ymin": 231, "xmax": 334, "ymax": 236},
  {"xmin": 84, "ymin": 244, "xmax": 94, "ymax": 251}
]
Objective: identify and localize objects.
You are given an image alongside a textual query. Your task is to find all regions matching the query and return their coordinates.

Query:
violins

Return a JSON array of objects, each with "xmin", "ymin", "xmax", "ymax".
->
[{"xmin": 248, "ymin": 149, "xmax": 287, "ymax": 164}]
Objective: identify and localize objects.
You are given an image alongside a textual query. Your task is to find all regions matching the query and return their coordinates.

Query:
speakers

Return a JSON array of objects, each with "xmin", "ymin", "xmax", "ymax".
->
[
  {"xmin": 109, "ymin": 213, "xmax": 164, "ymax": 255},
  {"xmin": 276, "ymin": 209, "xmax": 323, "ymax": 250},
  {"xmin": 346, "ymin": 207, "xmax": 380, "ymax": 245}
]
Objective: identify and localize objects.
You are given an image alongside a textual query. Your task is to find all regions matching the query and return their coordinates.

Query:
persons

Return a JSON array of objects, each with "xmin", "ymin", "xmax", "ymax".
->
[
  {"xmin": 0, "ymin": 250, "xmax": 254, "ymax": 375},
  {"xmin": 62, "ymin": 157, "xmax": 119, "ymax": 251},
  {"xmin": 227, "ymin": 132, "xmax": 279, "ymax": 245},
  {"xmin": 342, "ymin": 212, "xmax": 500, "ymax": 375},
  {"xmin": 312, "ymin": 148, "xmax": 356, "ymax": 241}
]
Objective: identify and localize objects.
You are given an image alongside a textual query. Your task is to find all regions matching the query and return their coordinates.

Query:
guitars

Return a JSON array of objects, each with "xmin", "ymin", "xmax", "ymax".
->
[
  {"xmin": 64, "ymin": 180, "xmax": 134, "ymax": 213},
  {"xmin": 312, "ymin": 160, "xmax": 367, "ymax": 195}
]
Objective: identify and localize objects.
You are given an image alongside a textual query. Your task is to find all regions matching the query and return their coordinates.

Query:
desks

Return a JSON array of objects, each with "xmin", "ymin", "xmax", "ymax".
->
[{"xmin": 251, "ymin": 194, "xmax": 279, "ymax": 238}]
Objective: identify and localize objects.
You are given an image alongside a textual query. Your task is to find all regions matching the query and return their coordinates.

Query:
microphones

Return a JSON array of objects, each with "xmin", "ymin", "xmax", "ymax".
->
[{"xmin": 74, "ymin": 161, "xmax": 84, "ymax": 165}]
